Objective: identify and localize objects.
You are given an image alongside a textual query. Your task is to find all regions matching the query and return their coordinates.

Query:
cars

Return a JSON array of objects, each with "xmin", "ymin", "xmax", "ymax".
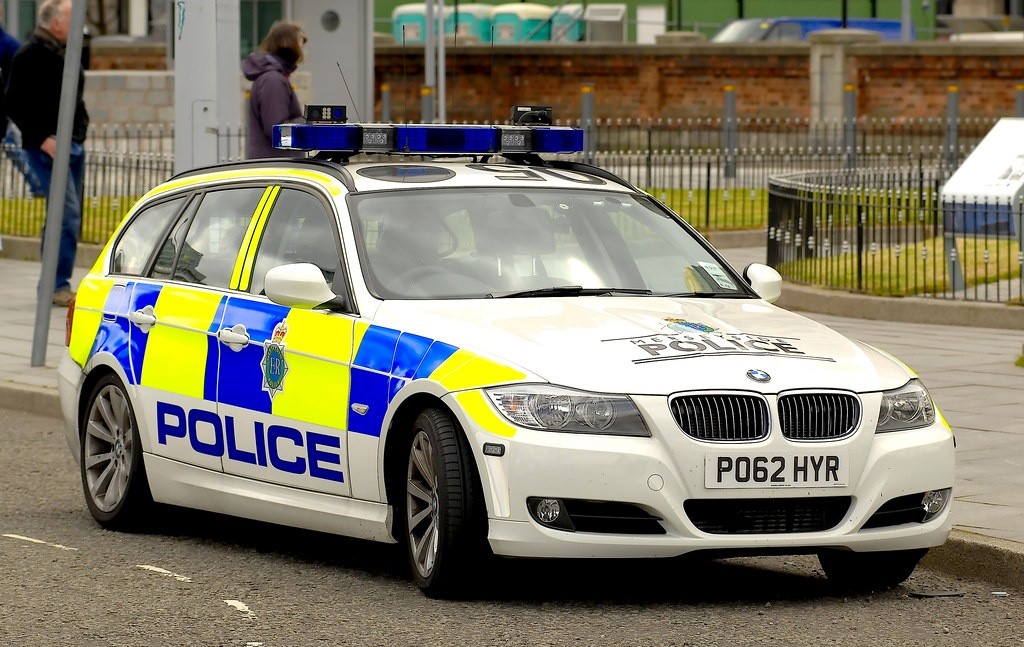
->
[{"xmin": 56, "ymin": 104, "xmax": 958, "ymax": 599}]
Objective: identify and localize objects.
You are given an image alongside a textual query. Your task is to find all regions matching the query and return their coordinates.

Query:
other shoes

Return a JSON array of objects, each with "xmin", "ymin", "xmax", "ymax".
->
[{"xmin": 52, "ymin": 289, "xmax": 76, "ymax": 305}]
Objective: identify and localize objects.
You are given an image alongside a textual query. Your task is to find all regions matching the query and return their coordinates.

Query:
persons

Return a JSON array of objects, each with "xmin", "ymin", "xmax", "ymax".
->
[
  {"xmin": 241, "ymin": 21, "xmax": 310, "ymax": 160},
  {"xmin": 0, "ymin": 0, "xmax": 90, "ymax": 307}
]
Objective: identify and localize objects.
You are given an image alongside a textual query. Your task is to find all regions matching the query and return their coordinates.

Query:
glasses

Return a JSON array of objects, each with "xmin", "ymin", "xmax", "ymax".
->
[{"xmin": 303, "ymin": 37, "xmax": 307, "ymax": 44}]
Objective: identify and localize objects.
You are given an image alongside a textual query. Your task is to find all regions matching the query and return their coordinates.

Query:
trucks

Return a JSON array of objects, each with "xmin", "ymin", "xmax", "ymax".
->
[{"xmin": 709, "ymin": 17, "xmax": 915, "ymax": 43}]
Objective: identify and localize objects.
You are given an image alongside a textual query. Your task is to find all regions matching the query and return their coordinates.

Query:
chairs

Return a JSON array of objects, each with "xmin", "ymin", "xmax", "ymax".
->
[{"xmin": 220, "ymin": 209, "xmax": 569, "ymax": 301}]
